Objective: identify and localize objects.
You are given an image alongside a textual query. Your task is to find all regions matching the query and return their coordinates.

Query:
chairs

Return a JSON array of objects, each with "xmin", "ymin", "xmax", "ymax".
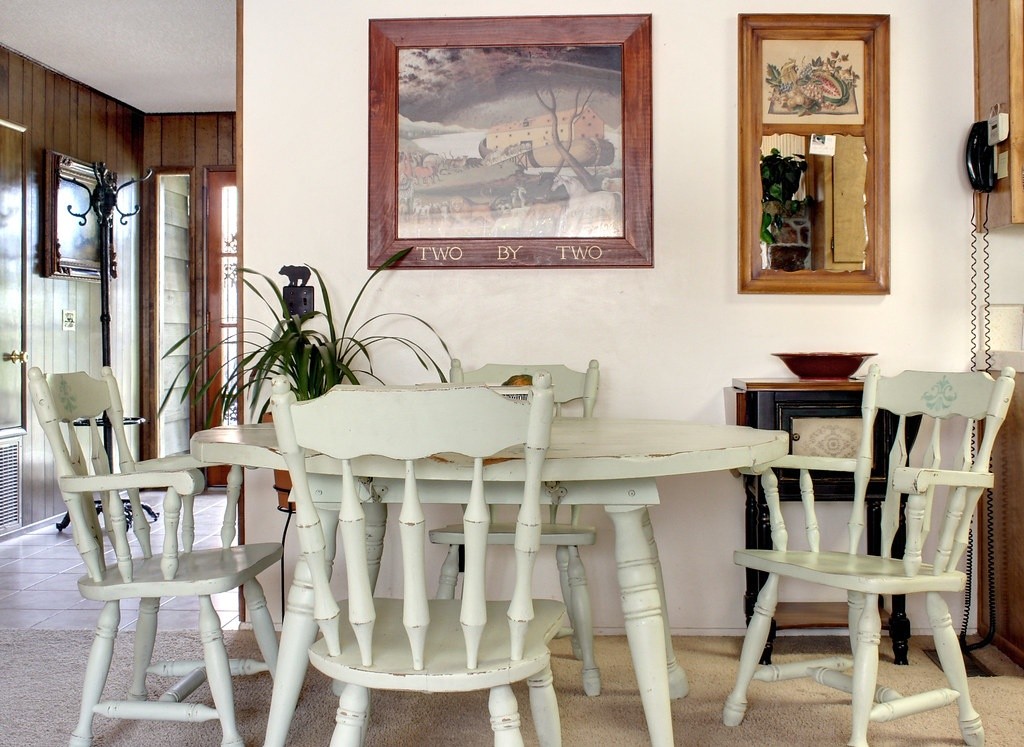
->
[
  {"xmin": 26, "ymin": 363, "xmax": 283, "ymax": 745},
  {"xmin": 722, "ymin": 361, "xmax": 1017, "ymax": 746},
  {"xmin": 426, "ymin": 357, "xmax": 603, "ymax": 699},
  {"xmin": 263, "ymin": 368, "xmax": 570, "ymax": 747}
]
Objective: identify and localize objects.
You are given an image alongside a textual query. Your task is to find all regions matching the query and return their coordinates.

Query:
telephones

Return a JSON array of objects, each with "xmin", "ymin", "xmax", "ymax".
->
[{"xmin": 965, "ymin": 120, "xmax": 997, "ymax": 194}]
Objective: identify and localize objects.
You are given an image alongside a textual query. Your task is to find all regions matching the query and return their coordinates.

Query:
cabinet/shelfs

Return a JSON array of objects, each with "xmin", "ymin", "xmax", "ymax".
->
[{"xmin": 732, "ymin": 376, "xmax": 923, "ymax": 665}]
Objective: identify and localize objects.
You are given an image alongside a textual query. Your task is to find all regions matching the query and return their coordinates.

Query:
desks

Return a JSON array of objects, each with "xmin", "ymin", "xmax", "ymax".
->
[{"xmin": 185, "ymin": 414, "xmax": 787, "ymax": 746}]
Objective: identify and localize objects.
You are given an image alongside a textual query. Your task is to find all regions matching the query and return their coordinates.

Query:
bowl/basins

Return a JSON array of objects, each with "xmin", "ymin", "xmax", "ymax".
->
[{"xmin": 771, "ymin": 352, "xmax": 879, "ymax": 381}]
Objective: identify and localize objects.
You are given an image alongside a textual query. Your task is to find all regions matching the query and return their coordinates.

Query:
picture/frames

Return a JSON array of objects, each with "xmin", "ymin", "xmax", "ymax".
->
[
  {"xmin": 38, "ymin": 147, "xmax": 117, "ymax": 283},
  {"xmin": 366, "ymin": 12, "xmax": 656, "ymax": 269}
]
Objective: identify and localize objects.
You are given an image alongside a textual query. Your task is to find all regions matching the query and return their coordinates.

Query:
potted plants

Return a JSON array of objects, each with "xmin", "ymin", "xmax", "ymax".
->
[
  {"xmin": 157, "ymin": 245, "xmax": 452, "ymax": 512},
  {"xmin": 759, "ymin": 147, "xmax": 815, "ymax": 244}
]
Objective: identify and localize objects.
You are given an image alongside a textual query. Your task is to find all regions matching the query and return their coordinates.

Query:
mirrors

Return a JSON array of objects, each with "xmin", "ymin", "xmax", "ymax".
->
[{"xmin": 759, "ymin": 133, "xmax": 869, "ymax": 273}]
